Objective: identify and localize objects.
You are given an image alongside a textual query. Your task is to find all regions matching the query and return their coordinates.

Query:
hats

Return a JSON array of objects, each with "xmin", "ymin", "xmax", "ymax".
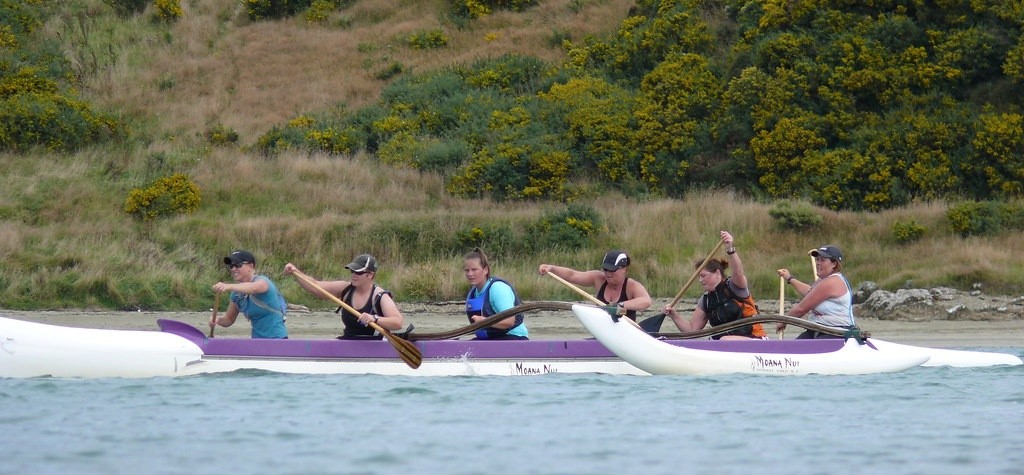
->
[
  {"xmin": 344, "ymin": 255, "xmax": 377, "ymax": 273},
  {"xmin": 224, "ymin": 250, "xmax": 256, "ymax": 265},
  {"xmin": 601, "ymin": 250, "xmax": 628, "ymax": 270},
  {"xmin": 811, "ymin": 245, "xmax": 842, "ymax": 262}
]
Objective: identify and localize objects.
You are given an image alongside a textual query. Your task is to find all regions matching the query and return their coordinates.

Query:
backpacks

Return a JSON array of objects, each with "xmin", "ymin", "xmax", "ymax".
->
[{"xmin": 236, "ymin": 275, "xmax": 287, "ymax": 323}]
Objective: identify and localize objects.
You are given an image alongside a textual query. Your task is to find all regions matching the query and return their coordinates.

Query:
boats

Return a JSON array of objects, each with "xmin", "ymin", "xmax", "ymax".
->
[{"xmin": 0, "ymin": 301, "xmax": 1023, "ymax": 379}]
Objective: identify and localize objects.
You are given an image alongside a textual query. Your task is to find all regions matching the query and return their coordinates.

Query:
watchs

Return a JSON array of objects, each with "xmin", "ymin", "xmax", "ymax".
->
[
  {"xmin": 374, "ymin": 315, "xmax": 378, "ymax": 323},
  {"xmin": 619, "ymin": 302, "xmax": 624, "ymax": 309}
]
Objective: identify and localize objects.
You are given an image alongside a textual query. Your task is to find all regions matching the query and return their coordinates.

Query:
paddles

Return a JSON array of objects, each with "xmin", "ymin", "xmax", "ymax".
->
[
  {"xmin": 543, "ymin": 268, "xmax": 667, "ymax": 341},
  {"xmin": 208, "ymin": 290, "xmax": 221, "ymax": 338},
  {"xmin": 778, "ymin": 270, "xmax": 785, "ymax": 339},
  {"xmin": 808, "ymin": 248, "xmax": 820, "ymax": 281},
  {"xmin": 637, "ymin": 239, "xmax": 723, "ymax": 333},
  {"xmin": 290, "ymin": 269, "xmax": 423, "ymax": 370}
]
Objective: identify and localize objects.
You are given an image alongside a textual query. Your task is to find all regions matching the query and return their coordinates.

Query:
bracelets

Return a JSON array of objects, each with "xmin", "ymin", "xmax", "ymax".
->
[
  {"xmin": 726, "ymin": 247, "xmax": 736, "ymax": 255},
  {"xmin": 787, "ymin": 275, "xmax": 795, "ymax": 284}
]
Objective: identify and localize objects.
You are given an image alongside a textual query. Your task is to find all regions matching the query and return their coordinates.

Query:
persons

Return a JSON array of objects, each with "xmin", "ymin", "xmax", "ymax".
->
[
  {"xmin": 777, "ymin": 246, "xmax": 855, "ymax": 340},
  {"xmin": 465, "ymin": 247, "xmax": 529, "ymax": 340},
  {"xmin": 283, "ymin": 254, "xmax": 403, "ymax": 341},
  {"xmin": 208, "ymin": 251, "xmax": 288, "ymax": 339},
  {"xmin": 661, "ymin": 231, "xmax": 770, "ymax": 340},
  {"xmin": 538, "ymin": 251, "xmax": 651, "ymax": 336}
]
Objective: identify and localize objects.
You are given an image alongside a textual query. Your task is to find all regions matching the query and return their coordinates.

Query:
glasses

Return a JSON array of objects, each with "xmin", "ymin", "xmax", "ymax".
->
[
  {"xmin": 603, "ymin": 268, "xmax": 622, "ymax": 272},
  {"xmin": 351, "ymin": 270, "xmax": 370, "ymax": 275},
  {"xmin": 230, "ymin": 262, "xmax": 250, "ymax": 269}
]
